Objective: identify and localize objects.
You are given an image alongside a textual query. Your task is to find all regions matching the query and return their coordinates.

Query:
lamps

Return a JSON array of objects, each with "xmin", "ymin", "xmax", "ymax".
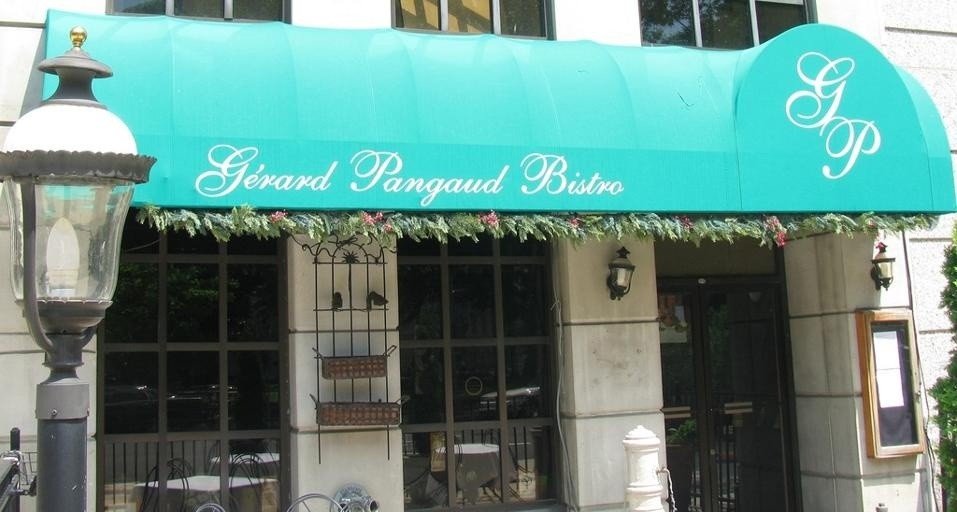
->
[
  {"xmin": 606, "ymin": 246, "xmax": 635, "ymax": 300},
  {"xmin": 871, "ymin": 246, "xmax": 895, "ymax": 291}
]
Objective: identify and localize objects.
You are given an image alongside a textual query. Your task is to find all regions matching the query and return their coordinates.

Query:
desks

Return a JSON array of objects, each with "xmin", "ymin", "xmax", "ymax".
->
[{"xmin": 431, "ymin": 444, "xmax": 499, "ymax": 504}]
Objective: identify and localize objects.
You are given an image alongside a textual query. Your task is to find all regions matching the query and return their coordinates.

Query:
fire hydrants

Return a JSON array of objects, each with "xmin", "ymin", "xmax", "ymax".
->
[{"xmin": 623, "ymin": 426, "xmax": 667, "ymax": 512}]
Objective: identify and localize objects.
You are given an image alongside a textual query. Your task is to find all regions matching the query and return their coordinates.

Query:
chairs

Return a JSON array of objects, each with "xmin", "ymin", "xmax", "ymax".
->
[
  {"xmin": 404, "ymin": 435, "xmax": 462, "ymax": 508},
  {"xmin": 139, "ymin": 439, "xmax": 280, "ymax": 512},
  {"xmin": 484, "ymin": 429, "xmax": 527, "ymax": 502},
  {"xmin": 285, "ymin": 493, "xmax": 344, "ymax": 512}
]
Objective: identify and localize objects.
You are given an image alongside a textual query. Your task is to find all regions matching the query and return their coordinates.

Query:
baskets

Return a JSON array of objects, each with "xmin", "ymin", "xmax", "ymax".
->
[
  {"xmin": 310, "ymin": 394, "xmax": 411, "ymax": 427},
  {"xmin": 313, "ymin": 345, "xmax": 397, "ymax": 379}
]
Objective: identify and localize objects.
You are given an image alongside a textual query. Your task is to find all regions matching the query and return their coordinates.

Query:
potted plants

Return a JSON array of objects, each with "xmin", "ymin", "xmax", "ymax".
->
[{"xmin": 667, "ymin": 420, "xmax": 698, "ymax": 512}]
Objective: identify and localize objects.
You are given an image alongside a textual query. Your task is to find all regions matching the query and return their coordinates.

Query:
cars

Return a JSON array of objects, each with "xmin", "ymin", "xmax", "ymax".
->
[
  {"xmin": 480, "ymin": 388, "xmax": 546, "ymax": 418},
  {"xmin": 105, "ymin": 376, "xmax": 239, "ymax": 432}
]
